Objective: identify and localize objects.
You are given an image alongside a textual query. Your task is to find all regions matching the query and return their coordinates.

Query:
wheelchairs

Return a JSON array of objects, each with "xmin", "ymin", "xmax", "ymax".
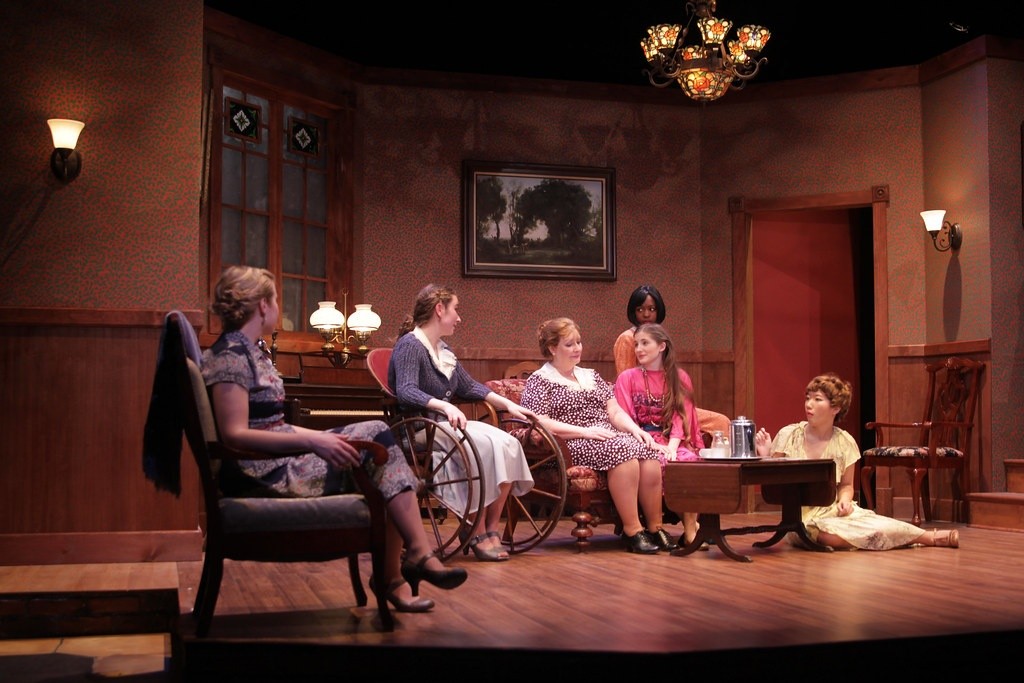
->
[{"xmin": 367, "ymin": 348, "xmax": 569, "ymax": 562}]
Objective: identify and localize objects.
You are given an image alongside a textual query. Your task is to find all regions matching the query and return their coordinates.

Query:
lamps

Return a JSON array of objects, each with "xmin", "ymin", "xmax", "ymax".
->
[
  {"xmin": 47, "ymin": 119, "xmax": 85, "ymax": 182},
  {"xmin": 641, "ymin": 0, "xmax": 771, "ymax": 103},
  {"xmin": 920, "ymin": 209, "xmax": 962, "ymax": 252},
  {"xmin": 309, "ymin": 289, "xmax": 382, "ymax": 369}
]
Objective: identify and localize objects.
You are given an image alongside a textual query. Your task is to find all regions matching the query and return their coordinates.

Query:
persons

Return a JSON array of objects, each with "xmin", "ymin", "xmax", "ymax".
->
[
  {"xmin": 388, "ymin": 285, "xmax": 539, "ymax": 562},
  {"xmin": 756, "ymin": 375, "xmax": 959, "ymax": 549},
  {"xmin": 200, "ymin": 266, "xmax": 468, "ymax": 611},
  {"xmin": 520, "ymin": 318, "xmax": 678, "ymax": 555},
  {"xmin": 614, "ymin": 323, "xmax": 709, "ymax": 550},
  {"xmin": 614, "ymin": 284, "xmax": 731, "ymax": 439}
]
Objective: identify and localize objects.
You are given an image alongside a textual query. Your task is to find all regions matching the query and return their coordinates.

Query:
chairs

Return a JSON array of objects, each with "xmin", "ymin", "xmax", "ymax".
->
[
  {"xmin": 862, "ymin": 354, "xmax": 985, "ymax": 528},
  {"xmin": 166, "ymin": 313, "xmax": 402, "ymax": 634}
]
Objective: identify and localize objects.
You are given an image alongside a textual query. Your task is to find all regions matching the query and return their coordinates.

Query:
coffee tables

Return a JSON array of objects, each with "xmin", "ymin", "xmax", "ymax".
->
[{"xmin": 662, "ymin": 455, "xmax": 837, "ymax": 561}]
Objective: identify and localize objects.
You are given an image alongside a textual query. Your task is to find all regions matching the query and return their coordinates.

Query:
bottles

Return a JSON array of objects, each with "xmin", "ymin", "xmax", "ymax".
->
[{"xmin": 709, "ymin": 430, "xmax": 732, "ymax": 458}]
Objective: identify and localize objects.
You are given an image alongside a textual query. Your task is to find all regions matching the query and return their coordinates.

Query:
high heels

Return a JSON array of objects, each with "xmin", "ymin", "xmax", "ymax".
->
[
  {"xmin": 369, "ymin": 575, "xmax": 435, "ymax": 612},
  {"xmin": 459, "ymin": 527, "xmax": 511, "ymax": 561},
  {"xmin": 401, "ymin": 553, "xmax": 468, "ymax": 596}
]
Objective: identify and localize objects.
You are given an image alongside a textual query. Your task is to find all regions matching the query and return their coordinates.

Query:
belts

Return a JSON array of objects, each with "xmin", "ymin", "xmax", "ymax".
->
[
  {"xmin": 644, "ymin": 426, "xmax": 663, "ymax": 431},
  {"xmin": 251, "ymin": 419, "xmax": 284, "ymax": 431}
]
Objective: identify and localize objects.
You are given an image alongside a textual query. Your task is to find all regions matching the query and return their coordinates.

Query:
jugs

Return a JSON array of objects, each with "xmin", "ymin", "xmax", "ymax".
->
[{"xmin": 729, "ymin": 416, "xmax": 757, "ymax": 458}]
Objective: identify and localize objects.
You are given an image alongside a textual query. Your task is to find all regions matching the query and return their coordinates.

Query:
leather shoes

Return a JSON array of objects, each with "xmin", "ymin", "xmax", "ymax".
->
[{"xmin": 622, "ymin": 528, "xmax": 678, "ymax": 555}]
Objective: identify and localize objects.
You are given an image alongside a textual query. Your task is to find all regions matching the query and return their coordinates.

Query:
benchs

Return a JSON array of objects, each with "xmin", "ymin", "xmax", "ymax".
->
[{"xmin": 483, "ymin": 378, "xmax": 732, "ymax": 552}]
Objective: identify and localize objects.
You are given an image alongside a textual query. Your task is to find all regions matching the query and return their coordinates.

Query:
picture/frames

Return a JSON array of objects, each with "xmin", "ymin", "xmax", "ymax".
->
[{"xmin": 461, "ymin": 159, "xmax": 617, "ymax": 282}]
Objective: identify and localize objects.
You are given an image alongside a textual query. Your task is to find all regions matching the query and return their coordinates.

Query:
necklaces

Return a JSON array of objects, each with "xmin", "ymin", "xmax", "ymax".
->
[
  {"xmin": 642, "ymin": 368, "xmax": 670, "ymax": 402},
  {"xmin": 642, "ymin": 365, "xmax": 666, "ymax": 428}
]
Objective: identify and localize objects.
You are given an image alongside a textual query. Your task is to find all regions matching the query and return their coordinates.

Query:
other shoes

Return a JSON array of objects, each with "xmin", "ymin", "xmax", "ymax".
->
[
  {"xmin": 678, "ymin": 533, "xmax": 710, "ymax": 551},
  {"xmin": 933, "ymin": 526, "xmax": 959, "ymax": 548}
]
